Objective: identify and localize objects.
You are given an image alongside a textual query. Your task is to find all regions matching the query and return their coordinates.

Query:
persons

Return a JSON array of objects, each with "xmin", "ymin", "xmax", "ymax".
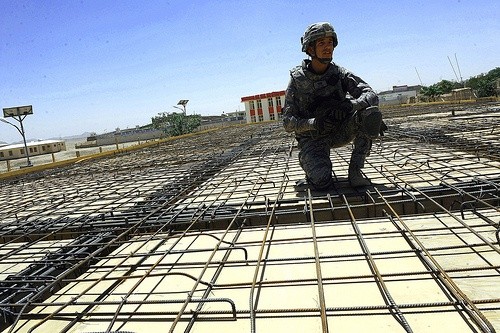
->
[{"xmin": 281, "ymin": 22, "xmax": 388, "ymax": 194}]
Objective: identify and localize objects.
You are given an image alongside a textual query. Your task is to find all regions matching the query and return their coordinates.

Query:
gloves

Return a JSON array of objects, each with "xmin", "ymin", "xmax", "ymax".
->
[
  {"xmin": 332, "ymin": 102, "xmax": 352, "ymax": 119},
  {"xmin": 317, "ymin": 116, "xmax": 341, "ymax": 135}
]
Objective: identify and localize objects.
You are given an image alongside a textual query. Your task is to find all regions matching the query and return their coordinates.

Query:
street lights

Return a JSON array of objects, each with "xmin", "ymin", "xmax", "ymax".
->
[
  {"xmin": 172, "ymin": 106, "xmax": 183, "ymax": 111},
  {"xmin": 1, "ymin": 118, "xmax": 22, "ymax": 135}
]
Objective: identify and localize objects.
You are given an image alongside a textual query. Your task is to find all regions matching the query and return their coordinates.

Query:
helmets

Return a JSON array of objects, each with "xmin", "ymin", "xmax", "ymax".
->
[{"xmin": 300, "ymin": 23, "xmax": 338, "ymax": 52}]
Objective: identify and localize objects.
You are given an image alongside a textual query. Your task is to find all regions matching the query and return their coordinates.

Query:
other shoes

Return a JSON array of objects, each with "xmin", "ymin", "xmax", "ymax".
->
[{"xmin": 348, "ymin": 164, "xmax": 366, "ymax": 189}]
